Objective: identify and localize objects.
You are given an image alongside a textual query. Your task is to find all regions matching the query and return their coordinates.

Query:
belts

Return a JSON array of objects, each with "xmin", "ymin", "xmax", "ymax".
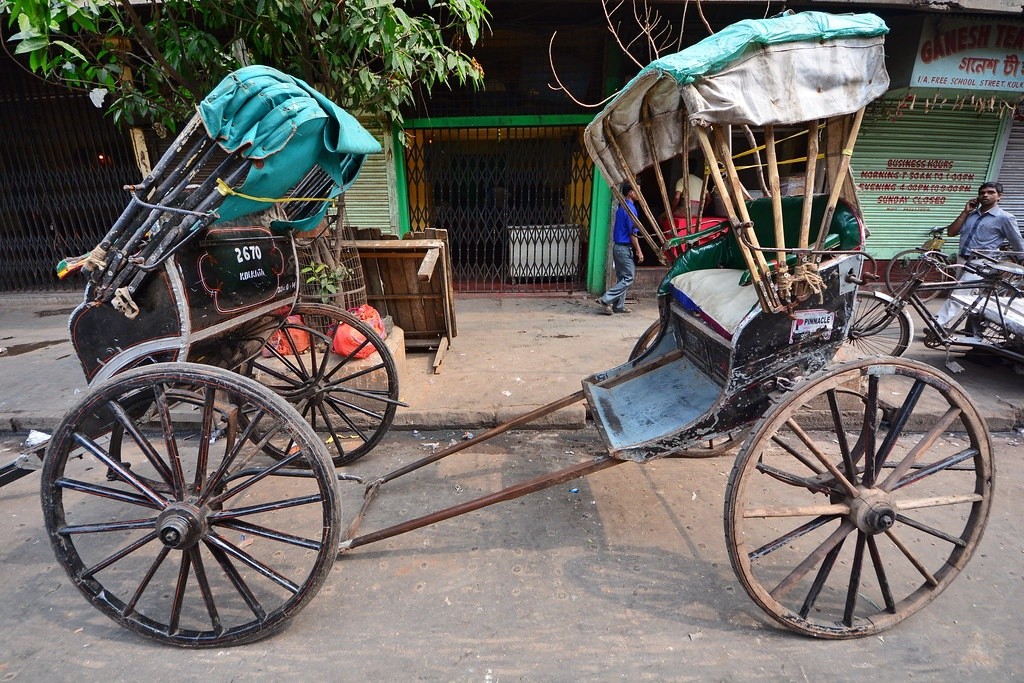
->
[
  {"xmin": 958, "ymin": 254, "xmax": 986, "ymax": 261},
  {"xmin": 615, "ymin": 241, "xmax": 632, "ymax": 246}
]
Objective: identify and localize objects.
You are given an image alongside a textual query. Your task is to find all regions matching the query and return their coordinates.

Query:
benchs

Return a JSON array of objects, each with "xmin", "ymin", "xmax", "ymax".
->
[
  {"xmin": 658, "ymin": 195, "xmax": 865, "ymax": 390},
  {"xmin": 68, "ymin": 184, "xmax": 299, "ymax": 416}
]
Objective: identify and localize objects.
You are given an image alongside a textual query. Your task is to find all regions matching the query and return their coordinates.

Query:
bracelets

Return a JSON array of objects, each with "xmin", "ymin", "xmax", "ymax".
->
[{"xmin": 958, "ymin": 217, "xmax": 964, "ymax": 222}]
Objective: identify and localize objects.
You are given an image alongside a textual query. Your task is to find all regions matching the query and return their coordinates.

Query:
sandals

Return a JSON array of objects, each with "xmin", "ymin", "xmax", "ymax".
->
[
  {"xmin": 611, "ymin": 305, "xmax": 632, "ymax": 313},
  {"xmin": 598, "ymin": 298, "xmax": 613, "ymax": 315}
]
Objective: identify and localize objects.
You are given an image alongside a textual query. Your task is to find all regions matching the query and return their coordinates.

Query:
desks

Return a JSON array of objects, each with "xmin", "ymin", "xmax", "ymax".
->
[{"xmin": 329, "ymin": 227, "xmax": 457, "ymax": 374}]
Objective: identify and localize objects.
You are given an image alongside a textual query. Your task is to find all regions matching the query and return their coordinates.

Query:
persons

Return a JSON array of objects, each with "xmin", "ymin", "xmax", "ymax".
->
[
  {"xmin": 933, "ymin": 182, "xmax": 1024, "ymax": 328},
  {"xmin": 657, "ymin": 158, "xmax": 711, "ymax": 227},
  {"xmin": 596, "ymin": 181, "xmax": 644, "ymax": 314}
]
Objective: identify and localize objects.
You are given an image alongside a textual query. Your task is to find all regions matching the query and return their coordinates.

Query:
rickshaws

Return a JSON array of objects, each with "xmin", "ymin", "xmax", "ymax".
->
[
  {"xmin": 334, "ymin": 9, "xmax": 998, "ymax": 641},
  {"xmin": 0, "ymin": 65, "xmax": 401, "ymax": 648}
]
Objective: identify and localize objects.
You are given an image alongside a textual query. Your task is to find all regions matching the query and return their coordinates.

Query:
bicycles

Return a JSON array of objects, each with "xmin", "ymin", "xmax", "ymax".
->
[{"xmin": 834, "ymin": 227, "xmax": 1024, "ymax": 374}]
